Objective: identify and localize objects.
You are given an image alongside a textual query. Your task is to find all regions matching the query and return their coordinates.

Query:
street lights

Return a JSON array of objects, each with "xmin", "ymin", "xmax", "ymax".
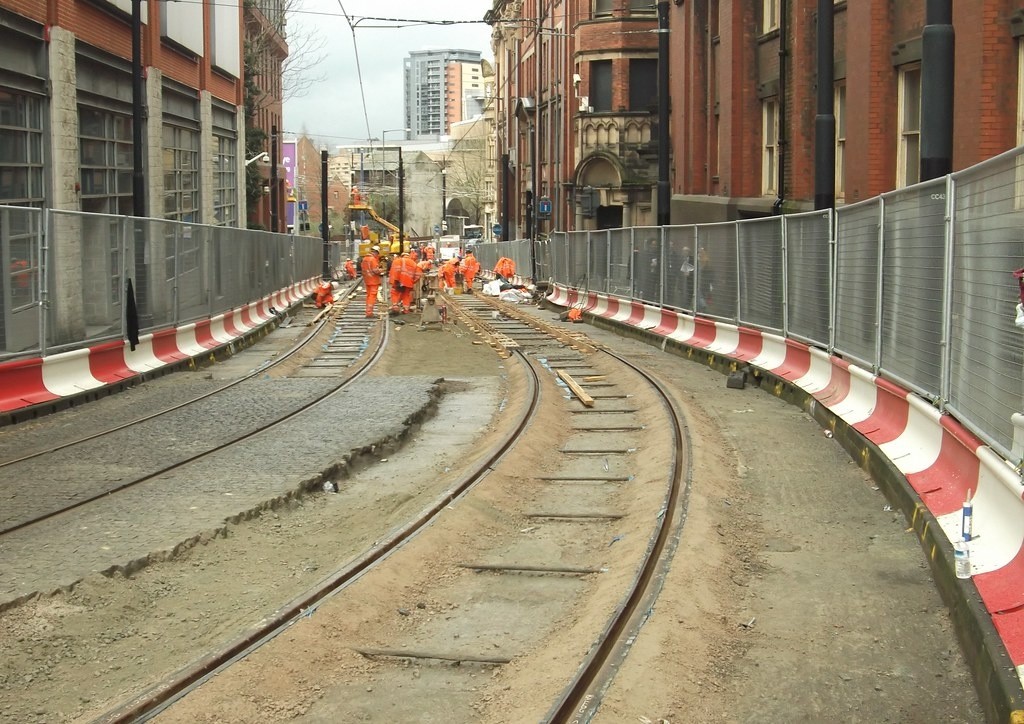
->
[{"xmin": 380, "ymin": 128, "xmax": 412, "ymax": 239}]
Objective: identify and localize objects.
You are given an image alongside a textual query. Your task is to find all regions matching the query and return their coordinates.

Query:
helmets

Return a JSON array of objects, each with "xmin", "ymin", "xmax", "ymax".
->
[
  {"xmin": 347, "ymin": 258, "xmax": 350, "ymax": 261},
  {"xmin": 465, "ymin": 248, "xmax": 472, "ymax": 253},
  {"xmin": 457, "ymin": 255, "xmax": 462, "ymax": 261},
  {"xmin": 371, "ymin": 246, "xmax": 381, "ymax": 254},
  {"xmin": 429, "ymin": 260, "xmax": 433, "ymax": 264},
  {"xmin": 331, "ymin": 282, "xmax": 339, "ymax": 290},
  {"xmin": 401, "ymin": 252, "xmax": 409, "ymax": 256}
]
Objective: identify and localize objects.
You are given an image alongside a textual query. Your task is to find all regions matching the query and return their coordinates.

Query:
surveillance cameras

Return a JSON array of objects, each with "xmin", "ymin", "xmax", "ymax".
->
[
  {"xmin": 263, "ymin": 156, "xmax": 270, "ymax": 163},
  {"xmin": 573, "ymin": 74, "xmax": 582, "ymax": 84}
]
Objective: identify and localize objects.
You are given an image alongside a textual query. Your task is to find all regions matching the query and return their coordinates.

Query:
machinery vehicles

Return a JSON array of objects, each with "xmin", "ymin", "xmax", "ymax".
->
[{"xmin": 345, "ymin": 202, "xmax": 435, "ymax": 275}]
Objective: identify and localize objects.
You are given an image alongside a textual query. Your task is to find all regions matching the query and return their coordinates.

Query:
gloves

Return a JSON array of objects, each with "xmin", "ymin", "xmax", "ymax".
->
[{"xmin": 395, "ymin": 280, "xmax": 399, "ymax": 286}]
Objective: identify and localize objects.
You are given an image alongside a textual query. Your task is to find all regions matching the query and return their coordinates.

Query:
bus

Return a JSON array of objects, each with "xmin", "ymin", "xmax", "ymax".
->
[
  {"xmin": 440, "ymin": 234, "xmax": 462, "ymax": 260},
  {"xmin": 463, "ymin": 224, "xmax": 486, "ymax": 253}
]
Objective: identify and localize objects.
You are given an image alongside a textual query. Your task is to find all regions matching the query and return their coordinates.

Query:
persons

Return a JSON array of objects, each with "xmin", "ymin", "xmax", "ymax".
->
[
  {"xmin": 438, "ymin": 265, "xmax": 446, "ymax": 288},
  {"xmin": 386, "ymin": 244, "xmax": 435, "ymax": 316},
  {"xmin": 461, "ymin": 251, "xmax": 476, "ymax": 293},
  {"xmin": 352, "ymin": 186, "xmax": 358, "ymax": 205},
  {"xmin": 446, "ymin": 242, "xmax": 452, "ymax": 248},
  {"xmin": 344, "ymin": 259, "xmax": 357, "ymax": 280},
  {"xmin": 312, "ymin": 283, "xmax": 334, "ymax": 308},
  {"xmin": 444, "ymin": 256, "xmax": 462, "ymax": 288},
  {"xmin": 362, "ymin": 247, "xmax": 384, "ymax": 318}
]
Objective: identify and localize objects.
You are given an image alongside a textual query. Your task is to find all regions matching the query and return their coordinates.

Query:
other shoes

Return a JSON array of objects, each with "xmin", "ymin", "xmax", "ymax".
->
[
  {"xmin": 321, "ymin": 303, "xmax": 326, "ymax": 307},
  {"xmin": 464, "ymin": 288, "xmax": 473, "ymax": 293},
  {"xmin": 367, "ymin": 313, "xmax": 379, "ymax": 317},
  {"xmin": 389, "ymin": 311, "xmax": 400, "ymax": 316}
]
